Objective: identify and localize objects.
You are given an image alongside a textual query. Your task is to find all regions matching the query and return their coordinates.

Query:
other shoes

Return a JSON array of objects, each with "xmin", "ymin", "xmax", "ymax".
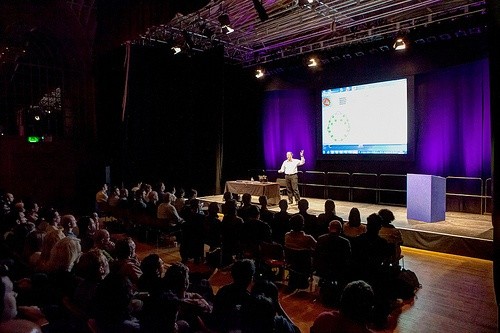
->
[
  {"xmin": 289, "ymin": 202, "xmax": 293, "ymax": 204},
  {"xmin": 295, "ymin": 201, "xmax": 299, "ymax": 204}
]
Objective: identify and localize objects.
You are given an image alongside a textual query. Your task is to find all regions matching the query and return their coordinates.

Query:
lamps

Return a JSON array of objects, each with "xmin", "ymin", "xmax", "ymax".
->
[{"xmin": 217, "ymin": 14, "xmax": 234, "ymax": 35}]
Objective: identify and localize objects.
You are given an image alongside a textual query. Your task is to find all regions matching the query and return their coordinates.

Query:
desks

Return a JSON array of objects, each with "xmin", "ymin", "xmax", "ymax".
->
[{"xmin": 223, "ymin": 180, "xmax": 281, "ymax": 206}]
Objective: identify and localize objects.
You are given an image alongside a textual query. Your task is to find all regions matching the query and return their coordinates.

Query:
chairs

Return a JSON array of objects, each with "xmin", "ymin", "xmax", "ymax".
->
[
  {"xmin": 93, "ymin": 202, "xmax": 312, "ymax": 294},
  {"xmin": 276, "ymin": 178, "xmax": 286, "ymax": 196}
]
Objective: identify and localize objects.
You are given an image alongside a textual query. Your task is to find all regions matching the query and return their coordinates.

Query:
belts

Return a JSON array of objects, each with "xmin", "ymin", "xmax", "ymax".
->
[{"xmin": 285, "ymin": 173, "xmax": 297, "ymax": 176}]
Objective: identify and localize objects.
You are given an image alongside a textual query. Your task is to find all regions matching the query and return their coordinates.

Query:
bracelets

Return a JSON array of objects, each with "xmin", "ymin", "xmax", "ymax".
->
[{"xmin": 301, "ymin": 156, "xmax": 303, "ymax": 157}]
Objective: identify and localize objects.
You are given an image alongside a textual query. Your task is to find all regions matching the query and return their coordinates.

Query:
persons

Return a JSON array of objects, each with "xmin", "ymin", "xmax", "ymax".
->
[
  {"xmin": 95, "ymin": 181, "xmax": 402, "ymax": 306},
  {"xmin": 0, "ymin": 192, "xmax": 301, "ymax": 333},
  {"xmin": 310, "ymin": 280, "xmax": 375, "ymax": 333},
  {"xmin": 278, "ymin": 150, "xmax": 305, "ymax": 205}
]
